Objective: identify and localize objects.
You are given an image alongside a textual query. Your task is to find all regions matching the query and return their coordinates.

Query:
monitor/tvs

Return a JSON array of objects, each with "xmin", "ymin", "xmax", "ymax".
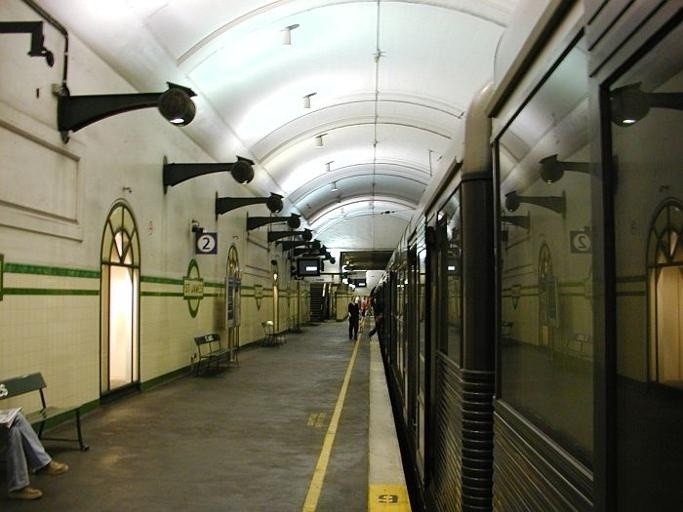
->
[
  {"xmin": 297, "ymin": 257, "xmax": 319, "ymax": 277},
  {"xmin": 358, "ymin": 283, "xmax": 366, "ymax": 287}
]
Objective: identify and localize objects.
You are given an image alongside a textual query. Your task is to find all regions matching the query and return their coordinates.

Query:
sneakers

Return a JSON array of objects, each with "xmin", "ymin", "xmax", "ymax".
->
[
  {"xmin": 32, "ymin": 459, "xmax": 68, "ymax": 477},
  {"xmin": 6, "ymin": 486, "xmax": 42, "ymax": 501}
]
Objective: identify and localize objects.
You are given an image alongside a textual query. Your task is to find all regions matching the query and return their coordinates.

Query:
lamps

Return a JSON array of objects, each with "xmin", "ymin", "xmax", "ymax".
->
[
  {"xmin": 498, "ymin": 80, "xmax": 683, "ymax": 238},
  {"xmin": 162, "ymin": 154, "xmax": 255, "ymax": 195},
  {"xmin": 246, "ymin": 212, "xmax": 300, "ymax": 232},
  {"xmin": 58, "ymin": 82, "xmax": 197, "ymax": 144},
  {"xmin": 280, "ymin": 27, "xmax": 291, "ymax": 46},
  {"xmin": 216, "ymin": 191, "xmax": 284, "ymax": 223},
  {"xmin": 267, "ymin": 228, "xmax": 336, "ymax": 265}
]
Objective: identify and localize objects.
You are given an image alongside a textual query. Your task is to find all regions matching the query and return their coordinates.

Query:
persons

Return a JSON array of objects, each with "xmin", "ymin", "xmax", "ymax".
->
[
  {"xmin": 353, "ymin": 295, "xmax": 372, "ymax": 316},
  {"xmin": 347, "ymin": 296, "xmax": 359, "ymax": 340},
  {"xmin": 367, "ymin": 302, "xmax": 382, "ymax": 338},
  {"xmin": 0, "ymin": 409, "xmax": 69, "ymax": 499}
]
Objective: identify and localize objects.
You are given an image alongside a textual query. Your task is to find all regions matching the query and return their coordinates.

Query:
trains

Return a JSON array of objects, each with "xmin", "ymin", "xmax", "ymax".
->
[{"xmin": 370, "ymin": 1, "xmax": 683, "ymax": 512}]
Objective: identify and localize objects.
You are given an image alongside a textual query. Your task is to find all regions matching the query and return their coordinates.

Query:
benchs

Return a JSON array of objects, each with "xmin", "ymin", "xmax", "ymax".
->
[
  {"xmin": 0, "ymin": 372, "xmax": 90, "ymax": 453},
  {"xmin": 262, "ymin": 321, "xmax": 287, "ymax": 346},
  {"xmin": 194, "ymin": 334, "xmax": 240, "ymax": 377}
]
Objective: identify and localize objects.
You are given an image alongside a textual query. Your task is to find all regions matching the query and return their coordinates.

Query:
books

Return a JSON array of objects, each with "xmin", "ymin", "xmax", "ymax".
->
[{"xmin": 0, "ymin": 406, "xmax": 23, "ymax": 429}]
